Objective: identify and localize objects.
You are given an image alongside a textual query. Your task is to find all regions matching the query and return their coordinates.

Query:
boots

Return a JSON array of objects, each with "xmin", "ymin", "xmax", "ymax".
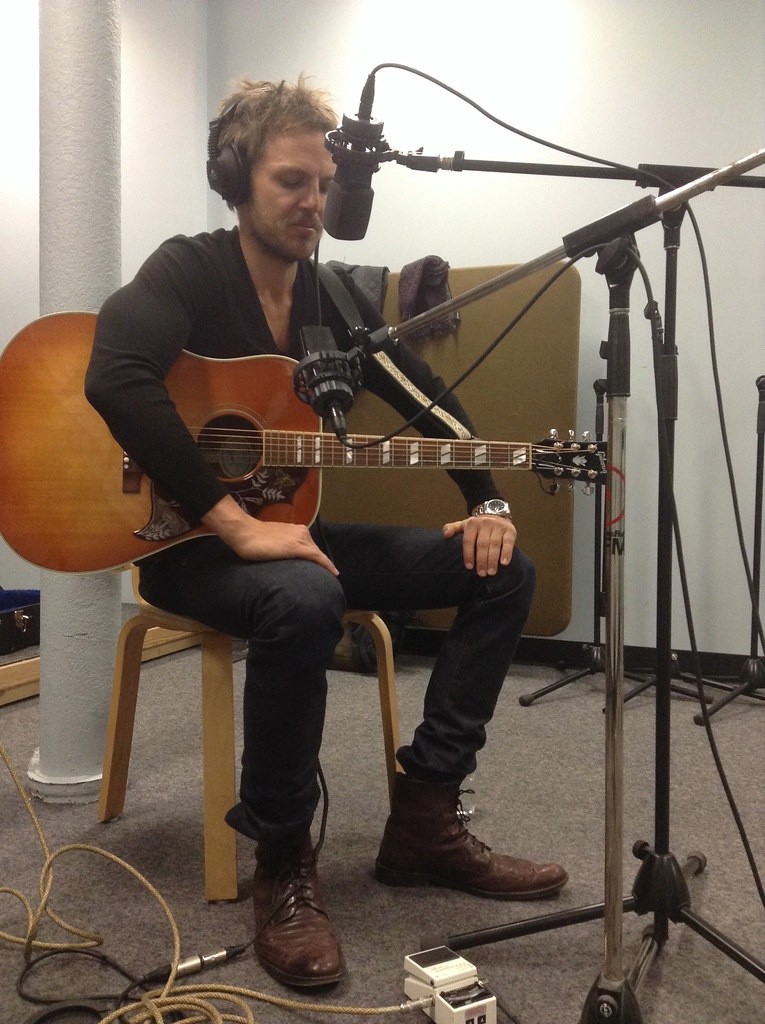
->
[
  {"xmin": 376, "ymin": 771, "xmax": 568, "ymax": 899},
  {"xmin": 223, "ymin": 801, "xmax": 343, "ymax": 988}
]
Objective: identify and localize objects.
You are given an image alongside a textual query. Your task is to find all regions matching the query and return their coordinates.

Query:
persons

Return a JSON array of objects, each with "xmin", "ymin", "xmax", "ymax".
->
[{"xmin": 83, "ymin": 82, "xmax": 569, "ymax": 987}]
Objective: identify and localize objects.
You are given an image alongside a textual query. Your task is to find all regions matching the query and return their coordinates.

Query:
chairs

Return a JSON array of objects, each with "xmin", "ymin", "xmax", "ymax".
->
[{"xmin": 96, "ymin": 561, "xmax": 404, "ymax": 903}]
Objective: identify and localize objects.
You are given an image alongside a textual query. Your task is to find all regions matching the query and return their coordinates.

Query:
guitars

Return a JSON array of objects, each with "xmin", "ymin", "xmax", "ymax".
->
[{"xmin": 0, "ymin": 305, "xmax": 615, "ymax": 577}]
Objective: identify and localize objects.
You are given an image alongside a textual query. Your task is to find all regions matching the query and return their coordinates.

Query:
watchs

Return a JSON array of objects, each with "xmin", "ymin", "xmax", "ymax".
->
[{"xmin": 472, "ymin": 499, "xmax": 512, "ymax": 519}]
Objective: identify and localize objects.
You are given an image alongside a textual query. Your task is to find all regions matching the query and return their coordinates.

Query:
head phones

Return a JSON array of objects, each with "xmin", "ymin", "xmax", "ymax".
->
[{"xmin": 206, "ymin": 100, "xmax": 247, "ymax": 210}]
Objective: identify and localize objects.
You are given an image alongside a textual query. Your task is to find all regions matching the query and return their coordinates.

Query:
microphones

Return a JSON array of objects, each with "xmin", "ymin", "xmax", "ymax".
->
[
  {"xmin": 323, "ymin": 76, "xmax": 377, "ymax": 239},
  {"xmin": 299, "ymin": 323, "xmax": 356, "ymax": 442}
]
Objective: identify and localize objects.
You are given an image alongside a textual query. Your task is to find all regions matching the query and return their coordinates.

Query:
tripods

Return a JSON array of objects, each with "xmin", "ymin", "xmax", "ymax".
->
[{"xmin": 357, "ymin": 148, "xmax": 764, "ymax": 1024}]
